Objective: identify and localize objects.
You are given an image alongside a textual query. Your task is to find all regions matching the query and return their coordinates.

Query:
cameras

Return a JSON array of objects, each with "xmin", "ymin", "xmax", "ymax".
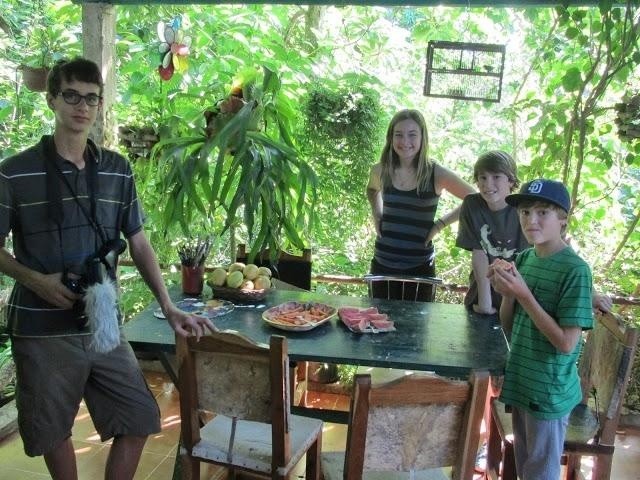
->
[{"xmin": 61, "ymin": 239, "xmax": 127, "ymax": 330}]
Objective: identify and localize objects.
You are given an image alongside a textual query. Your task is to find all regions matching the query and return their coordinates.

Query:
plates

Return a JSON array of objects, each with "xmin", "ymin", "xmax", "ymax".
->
[
  {"xmin": 338, "ymin": 307, "xmax": 398, "ymax": 334},
  {"xmin": 261, "ymin": 300, "xmax": 338, "ymax": 331}
]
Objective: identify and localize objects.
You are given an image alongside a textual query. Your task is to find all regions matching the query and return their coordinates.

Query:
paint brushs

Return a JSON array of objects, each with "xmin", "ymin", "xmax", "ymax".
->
[{"xmin": 176, "ymin": 232, "xmax": 219, "ymax": 267}]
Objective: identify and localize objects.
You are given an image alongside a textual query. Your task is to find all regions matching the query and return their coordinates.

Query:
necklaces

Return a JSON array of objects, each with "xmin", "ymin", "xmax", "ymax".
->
[{"xmin": 395, "ymin": 168, "xmax": 416, "ymax": 188}]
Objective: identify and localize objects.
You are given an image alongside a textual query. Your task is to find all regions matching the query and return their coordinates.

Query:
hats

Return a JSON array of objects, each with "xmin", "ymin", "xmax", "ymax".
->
[{"xmin": 504, "ymin": 178, "xmax": 570, "ymax": 213}]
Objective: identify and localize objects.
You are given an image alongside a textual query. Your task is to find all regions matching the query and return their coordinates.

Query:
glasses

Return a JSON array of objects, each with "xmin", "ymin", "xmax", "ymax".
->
[{"xmin": 54, "ymin": 86, "xmax": 103, "ymax": 106}]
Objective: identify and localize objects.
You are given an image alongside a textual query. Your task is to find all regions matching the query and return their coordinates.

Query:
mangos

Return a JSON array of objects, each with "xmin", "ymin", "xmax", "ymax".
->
[
  {"xmin": 226, "ymin": 271, "xmax": 244, "ymax": 289},
  {"xmin": 211, "ymin": 269, "xmax": 227, "ymax": 287},
  {"xmin": 240, "ymin": 278, "xmax": 254, "ymax": 290},
  {"xmin": 243, "ymin": 264, "xmax": 259, "ymax": 281},
  {"xmin": 252, "ymin": 275, "xmax": 271, "ymax": 291},
  {"xmin": 258, "ymin": 266, "xmax": 272, "ymax": 279},
  {"xmin": 229, "ymin": 262, "xmax": 245, "ymax": 274}
]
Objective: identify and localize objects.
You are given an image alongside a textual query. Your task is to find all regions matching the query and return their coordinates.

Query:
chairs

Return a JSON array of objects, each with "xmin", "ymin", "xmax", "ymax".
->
[
  {"xmin": 485, "ymin": 300, "xmax": 640, "ymax": 479},
  {"xmin": 318, "ymin": 367, "xmax": 489, "ymax": 480},
  {"xmin": 174, "ymin": 324, "xmax": 323, "ymax": 480},
  {"xmin": 363, "ymin": 273, "xmax": 442, "ymax": 303},
  {"xmin": 232, "ymin": 240, "xmax": 315, "ymax": 295}
]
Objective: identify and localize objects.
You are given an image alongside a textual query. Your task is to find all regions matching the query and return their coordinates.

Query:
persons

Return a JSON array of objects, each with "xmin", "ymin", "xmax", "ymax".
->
[
  {"xmin": 366, "ymin": 109, "xmax": 479, "ymax": 301},
  {"xmin": 1, "ymin": 57, "xmax": 219, "ymax": 480},
  {"xmin": 486, "ymin": 178, "xmax": 594, "ymax": 479},
  {"xmin": 456, "ymin": 150, "xmax": 533, "ymax": 480}
]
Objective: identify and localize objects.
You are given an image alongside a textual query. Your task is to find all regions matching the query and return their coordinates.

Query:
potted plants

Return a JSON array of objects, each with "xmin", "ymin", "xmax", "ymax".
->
[{"xmin": 21, "ymin": 29, "xmax": 64, "ymax": 92}]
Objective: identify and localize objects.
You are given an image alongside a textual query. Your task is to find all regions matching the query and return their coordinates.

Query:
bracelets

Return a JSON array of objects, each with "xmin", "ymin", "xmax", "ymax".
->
[{"xmin": 435, "ymin": 219, "xmax": 446, "ymax": 231}]
